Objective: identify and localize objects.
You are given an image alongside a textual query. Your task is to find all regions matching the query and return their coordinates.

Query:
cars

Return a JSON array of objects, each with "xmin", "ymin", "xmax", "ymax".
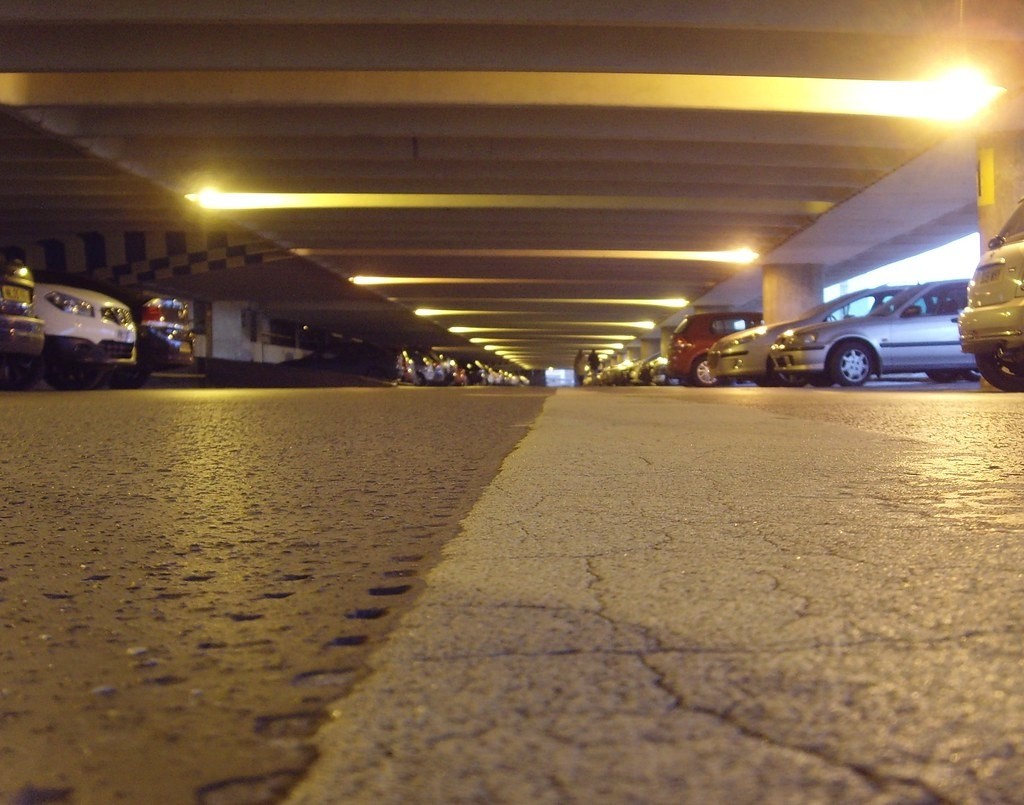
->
[
  {"xmin": 33, "ymin": 283, "xmax": 136, "ymax": 392},
  {"xmin": 708, "ymin": 286, "xmax": 915, "ymax": 386},
  {"xmin": 771, "ymin": 280, "xmax": 979, "ymax": 387},
  {"xmin": 34, "ymin": 270, "xmax": 194, "ymax": 392},
  {"xmin": 579, "ymin": 350, "xmax": 671, "ymax": 385},
  {"xmin": 958, "ymin": 198, "xmax": 1024, "ymax": 392},
  {"xmin": 275, "ymin": 342, "xmax": 530, "ymax": 387},
  {"xmin": 0, "ymin": 253, "xmax": 45, "ymax": 391}
]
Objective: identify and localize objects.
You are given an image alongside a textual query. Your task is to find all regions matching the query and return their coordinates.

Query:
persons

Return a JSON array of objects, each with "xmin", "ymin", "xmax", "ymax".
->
[{"xmin": 575, "ymin": 349, "xmax": 599, "ymax": 385}]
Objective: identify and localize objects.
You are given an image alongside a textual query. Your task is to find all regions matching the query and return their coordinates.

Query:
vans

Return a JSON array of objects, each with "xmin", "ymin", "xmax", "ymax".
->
[{"xmin": 669, "ymin": 312, "xmax": 764, "ymax": 387}]
